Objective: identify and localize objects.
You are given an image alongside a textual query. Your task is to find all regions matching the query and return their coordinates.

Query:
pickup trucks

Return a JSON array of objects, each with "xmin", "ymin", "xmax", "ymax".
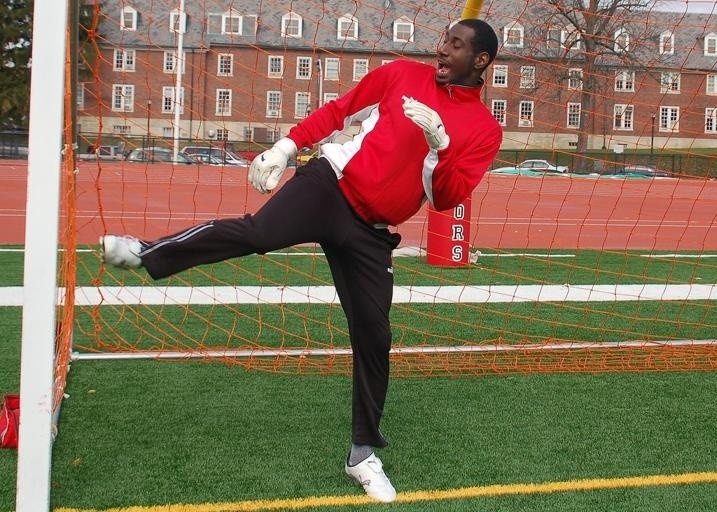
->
[{"xmin": 73, "ymin": 144, "xmax": 128, "ymax": 161}]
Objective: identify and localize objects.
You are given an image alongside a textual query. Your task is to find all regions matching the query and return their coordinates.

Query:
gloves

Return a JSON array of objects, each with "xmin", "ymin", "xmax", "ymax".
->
[
  {"xmin": 402, "ymin": 96, "xmax": 450, "ymax": 150},
  {"xmin": 247, "ymin": 137, "xmax": 298, "ymax": 194}
]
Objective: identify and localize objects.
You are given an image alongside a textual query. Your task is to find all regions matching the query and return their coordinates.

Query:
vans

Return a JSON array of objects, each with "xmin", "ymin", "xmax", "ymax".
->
[{"xmin": 180, "ymin": 145, "xmax": 248, "ymax": 165}]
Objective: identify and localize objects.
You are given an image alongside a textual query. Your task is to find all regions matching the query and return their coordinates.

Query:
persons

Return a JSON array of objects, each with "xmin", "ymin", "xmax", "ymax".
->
[{"xmin": 98, "ymin": 19, "xmax": 505, "ymax": 502}]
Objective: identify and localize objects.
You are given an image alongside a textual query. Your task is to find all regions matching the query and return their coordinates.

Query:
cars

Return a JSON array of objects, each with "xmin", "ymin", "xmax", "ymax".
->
[
  {"xmin": 187, "ymin": 154, "xmax": 222, "ymax": 164},
  {"xmin": 614, "ymin": 163, "xmax": 667, "ymax": 177},
  {"xmin": 515, "ymin": 159, "xmax": 569, "ymax": 173},
  {"xmin": 125, "ymin": 147, "xmax": 201, "ymax": 163},
  {"xmin": 484, "ymin": 166, "xmax": 540, "ymax": 178}
]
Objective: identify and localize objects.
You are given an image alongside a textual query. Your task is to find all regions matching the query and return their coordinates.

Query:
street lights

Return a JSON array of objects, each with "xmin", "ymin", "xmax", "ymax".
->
[
  {"xmin": 146, "ymin": 99, "xmax": 151, "ymax": 143},
  {"xmin": 649, "ymin": 112, "xmax": 655, "ymax": 159}
]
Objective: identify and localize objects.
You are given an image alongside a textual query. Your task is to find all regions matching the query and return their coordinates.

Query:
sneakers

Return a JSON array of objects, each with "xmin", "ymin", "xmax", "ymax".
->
[
  {"xmin": 99, "ymin": 233, "xmax": 143, "ymax": 269},
  {"xmin": 345, "ymin": 449, "xmax": 397, "ymax": 503}
]
